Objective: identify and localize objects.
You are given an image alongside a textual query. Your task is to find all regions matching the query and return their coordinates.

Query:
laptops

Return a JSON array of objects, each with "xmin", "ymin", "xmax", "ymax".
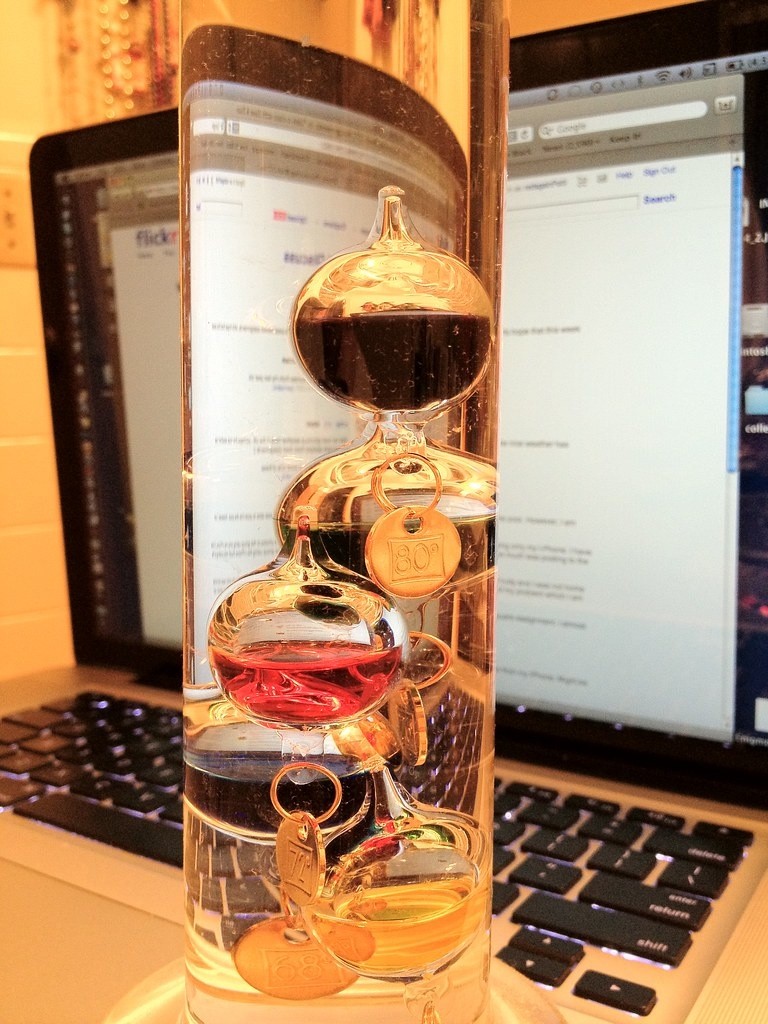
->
[
  {"xmin": 177, "ymin": 23, "xmax": 488, "ymax": 958},
  {"xmin": 1, "ymin": 1, "xmax": 768, "ymax": 1024}
]
